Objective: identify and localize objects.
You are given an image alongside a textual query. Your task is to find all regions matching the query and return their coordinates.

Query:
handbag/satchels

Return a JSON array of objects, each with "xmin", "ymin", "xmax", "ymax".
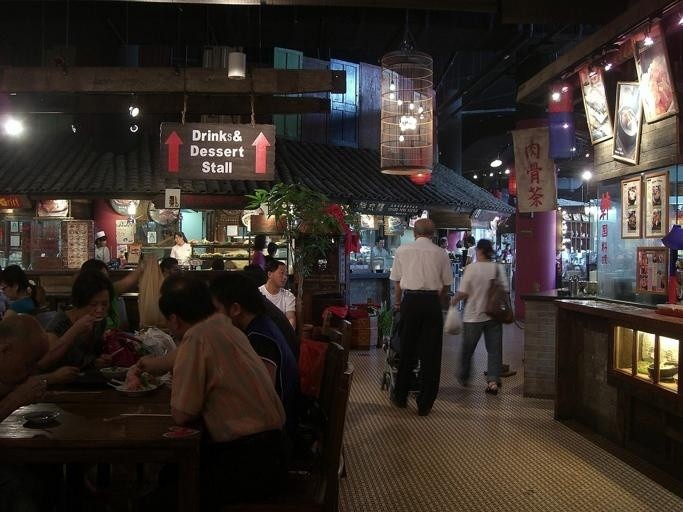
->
[
  {"xmin": 485, "ymin": 265, "xmax": 515, "ymax": 326},
  {"xmin": 101, "ymin": 326, "xmax": 143, "ymax": 367}
]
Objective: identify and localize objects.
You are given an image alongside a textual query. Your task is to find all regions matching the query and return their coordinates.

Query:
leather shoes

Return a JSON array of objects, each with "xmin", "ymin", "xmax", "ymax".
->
[
  {"xmin": 416, "ymin": 396, "xmax": 429, "ymax": 416},
  {"xmin": 389, "ymin": 392, "xmax": 408, "ymax": 408}
]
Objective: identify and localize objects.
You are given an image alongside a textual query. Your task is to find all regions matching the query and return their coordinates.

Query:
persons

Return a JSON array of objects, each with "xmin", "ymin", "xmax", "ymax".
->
[
  {"xmin": 141, "ymin": 272, "xmax": 286, "ymax": 442},
  {"xmin": 258, "ymin": 289, "xmax": 300, "ymax": 364},
  {"xmin": 265, "ymin": 242, "xmax": 278, "ymax": 267},
  {"xmin": 455, "ymin": 240, "xmax": 464, "ymax": 254},
  {"xmin": 440, "ymin": 237, "xmax": 448, "ymax": 256},
  {"xmin": 244, "ymin": 264, "xmax": 266, "ymax": 286},
  {"xmin": 81, "ymin": 259, "xmax": 147, "ymax": 328},
  {"xmin": 34, "ymin": 331, "xmax": 80, "ymax": 386},
  {"xmin": 160, "ymin": 258, "xmax": 184, "ymax": 274},
  {"xmin": 448, "ymin": 239, "xmax": 502, "ymax": 395},
  {"xmin": 257, "ymin": 261, "xmax": 296, "ymax": 331},
  {"xmin": 466, "ymin": 236, "xmax": 475, "ymax": 265},
  {"xmin": 170, "ymin": 232, "xmax": 191, "ymax": 266},
  {"xmin": 0, "ymin": 265, "xmax": 39, "ymax": 315},
  {"xmin": 44, "ymin": 270, "xmax": 116, "ymax": 378},
  {"xmin": 502, "ymin": 244, "xmax": 512, "ymax": 264},
  {"xmin": 389, "ymin": 220, "xmax": 453, "ymax": 417},
  {"xmin": 212, "ymin": 274, "xmax": 303, "ymax": 418},
  {"xmin": 95, "ymin": 231, "xmax": 108, "ymax": 267},
  {"xmin": 372, "ymin": 236, "xmax": 388, "ymax": 257},
  {"xmin": 1, "ymin": 312, "xmax": 47, "ymax": 421},
  {"xmin": 496, "ymin": 242, "xmax": 501, "ymax": 257},
  {"xmin": 253, "ymin": 235, "xmax": 266, "ymax": 267}
]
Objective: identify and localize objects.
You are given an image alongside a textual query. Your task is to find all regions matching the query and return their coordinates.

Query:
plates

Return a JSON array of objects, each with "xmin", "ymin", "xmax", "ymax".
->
[
  {"xmin": 116, "ymin": 384, "xmax": 156, "ymax": 397},
  {"xmin": 22, "ymin": 410, "xmax": 58, "ymax": 424}
]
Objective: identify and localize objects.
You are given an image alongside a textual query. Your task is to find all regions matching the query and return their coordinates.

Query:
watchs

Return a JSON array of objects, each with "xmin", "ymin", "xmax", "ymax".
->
[{"xmin": 41, "ymin": 374, "xmax": 48, "ymax": 385}]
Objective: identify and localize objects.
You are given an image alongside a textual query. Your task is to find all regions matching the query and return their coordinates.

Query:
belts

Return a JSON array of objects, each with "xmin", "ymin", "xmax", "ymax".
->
[{"xmin": 405, "ymin": 289, "xmax": 440, "ymax": 296}]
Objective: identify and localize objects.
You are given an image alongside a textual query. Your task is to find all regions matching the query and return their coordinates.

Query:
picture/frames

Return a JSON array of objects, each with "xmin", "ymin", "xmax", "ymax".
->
[
  {"xmin": 629, "ymin": 19, "xmax": 679, "ymax": 124},
  {"xmin": 621, "ymin": 176, "xmax": 641, "ymax": 238},
  {"xmin": 577, "ymin": 64, "xmax": 614, "ymax": 146},
  {"xmin": 642, "ymin": 170, "xmax": 670, "ymax": 238},
  {"xmin": 611, "ymin": 81, "xmax": 640, "ymax": 165},
  {"xmin": 636, "ymin": 246, "xmax": 669, "ymax": 295}
]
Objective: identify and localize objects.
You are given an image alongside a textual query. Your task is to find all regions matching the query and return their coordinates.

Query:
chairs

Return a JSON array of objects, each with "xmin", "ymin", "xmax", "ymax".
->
[
  {"xmin": 317, "ymin": 326, "xmax": 341, "ymax": 341},
  {"xmin": 322, "ymin": 310, "xmax": 348, "ymax": 344},
  {"xmin": 220, "ymin": 342, "xmax": 354, "ymax": 511}
]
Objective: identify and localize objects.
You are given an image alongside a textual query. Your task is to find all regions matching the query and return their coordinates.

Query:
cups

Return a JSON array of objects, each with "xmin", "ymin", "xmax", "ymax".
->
[
  {"xmin": 92, "ymin": 314, "xmax": 107, "ymax": 334},
  {"xmin": 31, "ymin": 375, "xmax": 47, "ymax": 399}
]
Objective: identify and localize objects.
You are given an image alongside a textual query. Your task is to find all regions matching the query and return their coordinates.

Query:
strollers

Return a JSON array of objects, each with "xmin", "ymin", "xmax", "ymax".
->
[{"xmin": 379, "ymin": 306, "xmax": 420, "ymax": 400}]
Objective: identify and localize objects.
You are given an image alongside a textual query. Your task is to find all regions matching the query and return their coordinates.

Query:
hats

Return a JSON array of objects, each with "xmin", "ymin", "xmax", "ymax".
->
[{"xmin": 95, "ymin": 230, "xmax": 106, "ymax": 240}]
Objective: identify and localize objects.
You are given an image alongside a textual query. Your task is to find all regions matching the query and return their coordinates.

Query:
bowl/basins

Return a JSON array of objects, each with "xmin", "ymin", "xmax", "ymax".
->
[
  {"xmin": 101, "ymin": 367, "xmax": 126, "ymax": 378},
  {"xmin": 647, "ymin": 361, "xmax": 679, "ymax": 376}
]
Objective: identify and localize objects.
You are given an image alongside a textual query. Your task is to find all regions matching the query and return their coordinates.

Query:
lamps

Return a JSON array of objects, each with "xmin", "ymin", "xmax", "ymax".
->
[
  {"xmin": 586, "ymin": 153, "xmax": 587, "ymax": 155},
  {"xmin": 504, "ymin": 169, "xmax": 510, "ymax": 175},
  {"xmin": 562, "ymin": 86, "xmax": 569, "ymax": 92},
  {"xmin": 499, "ymin": 172, "xmax": 501, "ymax": 175},
  {"xmin": 564, "ymin": 124, "xmax": 568, "ymax": 129},
  {"xmin": 128, "ymin": 105, "xmax": 140, "ymax": 117},
  {"xmin": 603, "ymin": 63, "xmax": 614, "ymax": 69},
  {"xmin": 130, "ymin": 124, "xmax": 138, "ymax": 133},
  {"xmin": 551, "ymin": 92, "xmax": 561, "ymax": 102},
  {"xmin": 482, "ymin": 173, "xmax": 486, "ymax": 176},
  {"xmin": 489, "ymin": 159, "xmax": 502, "ymax": 168},
  {"xmin": 228, "ymin": 50, "xmax": 247, "ymax": 81},
  {"xmin": 382, "ymin": 36, "xmax": 434, "ymax": 174},
  {"xmin": 472, "ymin": 174, "xmax": 479, "ymax": 179},
  {"xmin": 642, "ymin": 32, "xmax": 654, "ymax": 46},
  {"xmin": 71, "ymin": 125, "xmax": 77, "ymax": 133},
  {"xmin": 0, "ymin": 117, "xmax": 27, "ymax": 140},
  {"xmin": 490, "ymin": 173, "xmax": 494, "ymax": 177}
]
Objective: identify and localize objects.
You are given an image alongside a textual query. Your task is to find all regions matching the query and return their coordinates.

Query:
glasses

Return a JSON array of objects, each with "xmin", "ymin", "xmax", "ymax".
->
[{"xmin": 1, "ymin": 285, "xmax": 8, "ymax": 291}]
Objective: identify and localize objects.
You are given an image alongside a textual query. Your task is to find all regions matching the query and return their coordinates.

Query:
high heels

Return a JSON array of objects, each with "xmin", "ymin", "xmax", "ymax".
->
[{"xmin": 486, "ymin": 384, "xmax": 499, "ymax": 397}]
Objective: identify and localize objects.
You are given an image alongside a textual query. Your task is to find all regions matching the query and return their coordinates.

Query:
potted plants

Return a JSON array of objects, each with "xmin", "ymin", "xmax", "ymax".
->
[{"xmin": 243, "ymin": 180, "xmax": 356, "ymax": 297}]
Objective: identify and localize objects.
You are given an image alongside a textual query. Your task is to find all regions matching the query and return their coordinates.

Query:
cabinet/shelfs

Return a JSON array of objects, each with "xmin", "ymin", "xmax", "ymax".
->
[
  {"xmin": 183, "ymin": 239, "xmax": 288, "ymax": 275},
  {"xmin": 1, "ymin": 212, "xmax": 94, "ymax": 274},
  {"xmin": 555, "ymin": 296, "xmax": 683, "ymax": 496}
]
